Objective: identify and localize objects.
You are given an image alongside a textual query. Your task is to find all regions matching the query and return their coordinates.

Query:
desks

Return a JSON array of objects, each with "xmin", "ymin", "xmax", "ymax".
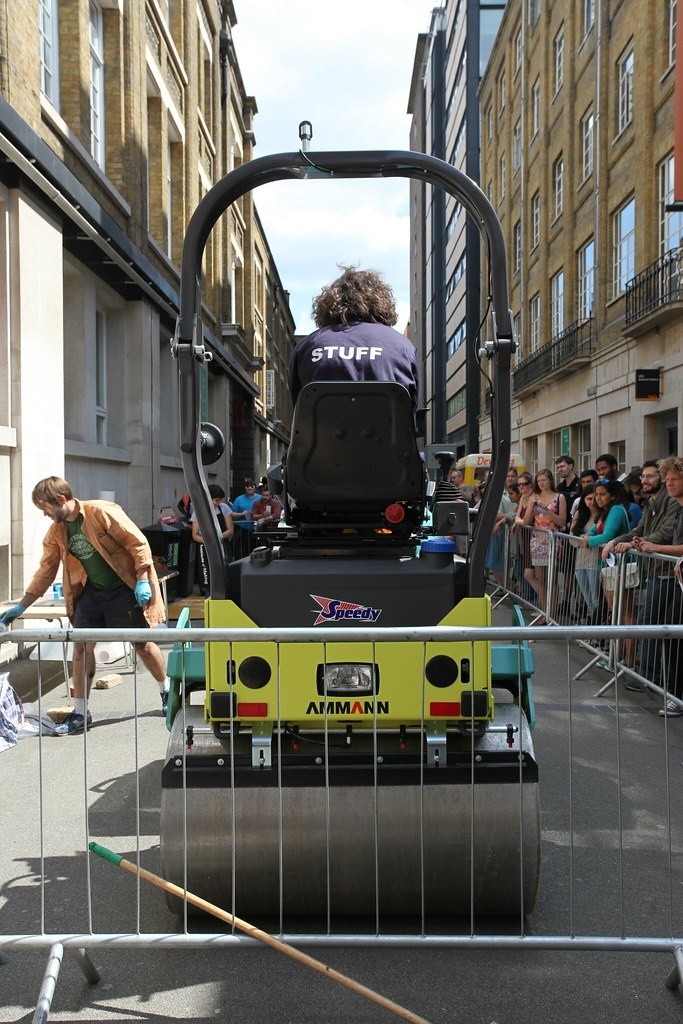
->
[{"xmin": 0, "ymin": 571, "xmax": 179, "ymax": 707}]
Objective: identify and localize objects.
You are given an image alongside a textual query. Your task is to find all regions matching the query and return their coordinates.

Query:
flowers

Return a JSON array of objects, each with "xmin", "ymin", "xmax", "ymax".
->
[{"xmin": 153, "ymin": 555, "xmax": 172, "ymax": 574}]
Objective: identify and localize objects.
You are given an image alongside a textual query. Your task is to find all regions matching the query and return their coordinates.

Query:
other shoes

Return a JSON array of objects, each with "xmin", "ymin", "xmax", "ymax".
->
[
  {"xmin": 493, "ymin": 592, "xmax": 548, "ymax": 625},
  {"xmin": 624, "ymin": 680, "xmax": 647, "ymax": 692},
  {"xmin": 596, "ymin": 658, "xmax": 625, "ymax": 672}
]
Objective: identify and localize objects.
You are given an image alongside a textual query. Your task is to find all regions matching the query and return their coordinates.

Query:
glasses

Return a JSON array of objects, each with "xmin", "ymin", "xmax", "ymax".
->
[
  {"xmin": 633, "ymin": 486, "xmax": 643, "ymax": 495},
  {"xmin": 596, "ymin": 479, "xmax": 610, "ymax": 484},
  {"xmin": 515, "ymin": 482, "xmax": 530, "ymax": 486},
  {"xmin": 639, "ymin": 474, "xmax": 656, "ymax": 480}
]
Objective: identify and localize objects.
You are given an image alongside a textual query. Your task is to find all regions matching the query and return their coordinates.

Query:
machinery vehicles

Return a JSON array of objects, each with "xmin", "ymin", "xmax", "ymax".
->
[{"xmin": 160, "ymin": 122, "xmax": 539, "ymax": 916}]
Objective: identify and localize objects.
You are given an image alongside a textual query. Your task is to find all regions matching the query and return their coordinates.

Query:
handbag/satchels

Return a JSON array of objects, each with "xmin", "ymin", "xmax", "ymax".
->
[{"xmin": 601, "ymin": 562, "xmax": 640, "ymax": 591}]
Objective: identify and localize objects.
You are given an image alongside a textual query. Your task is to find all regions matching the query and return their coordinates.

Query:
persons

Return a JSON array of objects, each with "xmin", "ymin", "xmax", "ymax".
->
[
  {"xmin": 451, "ymin": 454, "xmax": 683, "ymax": 717},
  {"xmin": 190, "ymin": 477, "xmax": 282, "ymax": 595},
  {"xmin": 0, "ymin": 476, "xmax": 171, "ymax": 736},
  {"xmin": 287, "ymin": 269, "xmax": 418, "ymax": 416}
]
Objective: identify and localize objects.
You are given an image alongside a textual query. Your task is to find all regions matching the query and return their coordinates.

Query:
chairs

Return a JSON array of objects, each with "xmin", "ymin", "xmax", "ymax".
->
[{"xmin": 279, "ymin": 379, "xmax": 428, "ymax": 542}]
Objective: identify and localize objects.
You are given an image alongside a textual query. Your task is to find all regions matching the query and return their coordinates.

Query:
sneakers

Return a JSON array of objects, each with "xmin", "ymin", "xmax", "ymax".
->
[
  {"xmin": 52, "ymin": 711, "xmax": 94, "ymax": 736},
  {"xmin": 659, "ymin": 700, "xmax": 682, "ymax": 716},
  {"xmin": 160, "ymin": 690, "xmax": 169, "ymax": 717}
]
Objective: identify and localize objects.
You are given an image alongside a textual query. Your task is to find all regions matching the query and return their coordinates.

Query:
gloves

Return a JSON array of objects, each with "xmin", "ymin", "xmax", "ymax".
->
[
  {"xmin": 0, "ymin": 605, "xmax": 26, "ymax": 627},
  {"xmin": 134, "ymin": 581, "xmax": 152, "ymax": 607}
]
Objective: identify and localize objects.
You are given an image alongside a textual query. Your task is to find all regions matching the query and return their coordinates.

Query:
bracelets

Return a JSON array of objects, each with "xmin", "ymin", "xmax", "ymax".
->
[
  {"xmin": 630, "ymin": 541, "xmax": 634, "ymax": 547},
  {"xmin": 514, "ymin": 522, "xmax": 518, "ymax": 526}
]
